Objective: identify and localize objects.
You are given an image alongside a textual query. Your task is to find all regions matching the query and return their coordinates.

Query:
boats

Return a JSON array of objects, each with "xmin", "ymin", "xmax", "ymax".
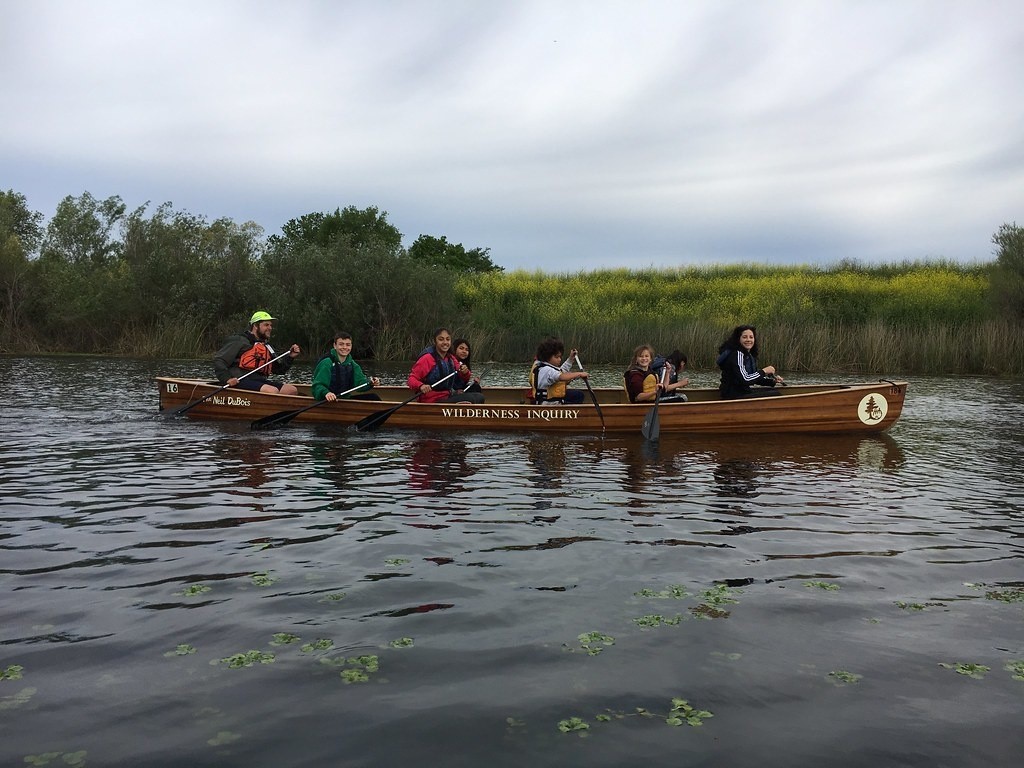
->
[{"xmin": 149, "ymin": 376, "xmax": 908, "ymax": 435}]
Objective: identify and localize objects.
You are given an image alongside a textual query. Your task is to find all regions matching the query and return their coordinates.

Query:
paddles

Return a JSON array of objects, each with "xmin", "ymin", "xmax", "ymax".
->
[
  {"xmin": 251, "ymin": 378, "xmax": 377, "ymax": 431},
  {"xmin": 640, "ymin": 362, "xmax": 668, "ymax": 442},
  {"xmin": 347, "ymin": 365, "xmax": 463, "ymax": 435},
  {"xmin": 169, "ymin": 348, "xmax": 298, "ymax": 421},
  {"xmin": 573, "ymin": 348, "xmax": 606, "ymax": 427},
  {"xmin": 460, "ymin": 368, "xmax": 493, "ymax": 393},
  {"xmin": 771, "ymin": 373, "xmax": 788, "ymax": 387}
]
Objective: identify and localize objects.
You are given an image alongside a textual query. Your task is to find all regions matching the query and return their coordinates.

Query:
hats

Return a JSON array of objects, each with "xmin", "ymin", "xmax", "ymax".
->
[{"xmin": 250, "ymin": 310, "xmax": 278, "ymax": 326}]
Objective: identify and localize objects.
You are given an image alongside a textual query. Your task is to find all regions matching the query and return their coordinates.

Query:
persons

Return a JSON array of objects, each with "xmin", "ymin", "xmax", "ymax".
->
[
  {"xmin": 622, "ymin": 345, "xmax": 689, "ymax": 404},
  {"xmin": 311, "ymin": 332, "xmax": 382, "ymax": 402},
  {"xmin": 528, "ymin": 338, "xmax": 589, "ymax": 405},
  {"xmin": 407, "ymin": 327, "xmax": 485, "ymax": 404},
  {"xmin": 716, "ymin": 325, "xmax": 784, "ymax": 401},
  {"xmin": 213, "ymin": 311, "xmax": 300, "ymax": 396}
]
